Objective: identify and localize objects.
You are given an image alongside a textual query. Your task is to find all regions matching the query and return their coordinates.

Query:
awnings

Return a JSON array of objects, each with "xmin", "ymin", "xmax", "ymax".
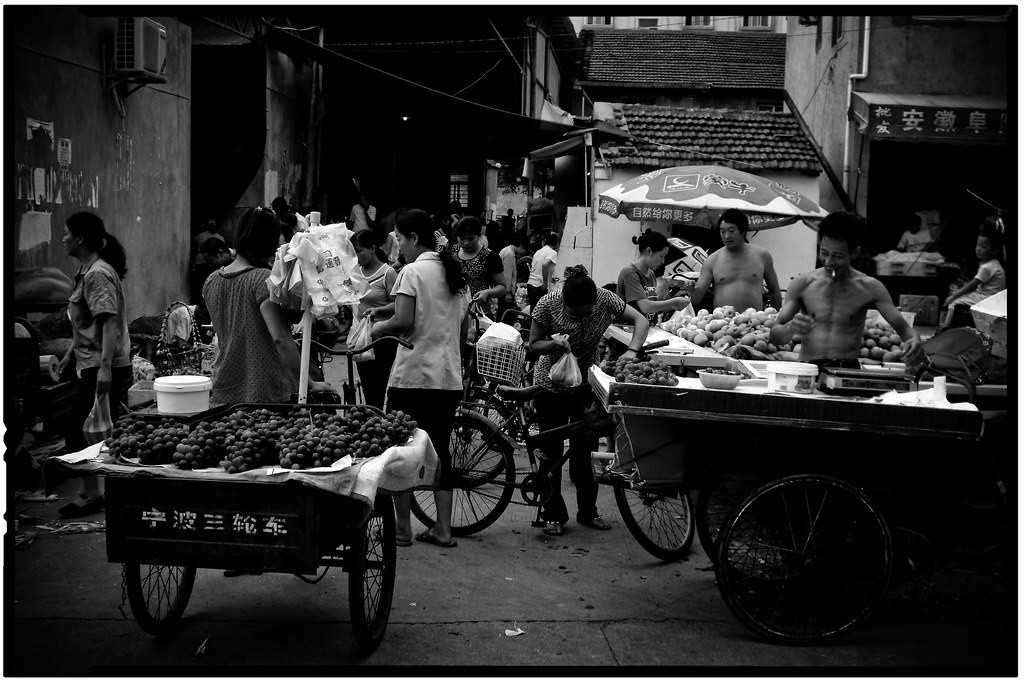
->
[{"xmin": 258, "ymin": 21, "xmax": 590, "ymax": 162}]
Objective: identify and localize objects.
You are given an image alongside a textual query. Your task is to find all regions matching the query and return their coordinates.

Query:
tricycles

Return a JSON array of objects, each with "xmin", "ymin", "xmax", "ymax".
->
[{"xmin": 101, "ymin": 335, "xmax": 415, "ymax": 658}]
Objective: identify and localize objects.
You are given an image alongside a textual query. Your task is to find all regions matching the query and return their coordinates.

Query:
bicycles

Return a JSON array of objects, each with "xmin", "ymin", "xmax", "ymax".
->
[{"xmin": 410, "ymin": 300, "xmax": 696, "ymax": 563}]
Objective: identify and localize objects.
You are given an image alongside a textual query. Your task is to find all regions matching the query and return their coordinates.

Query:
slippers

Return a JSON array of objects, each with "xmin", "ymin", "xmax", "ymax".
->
[
  {"xmin": 543, "ymin": 520, "xmax": 562, "ymax": 535},
  {"xmin": 415, "ymin": 528, "xmax": 458, "ymax": 547},
  {"xmin": 577, "ymin": 514, "xmax": 612, "ymax": 530},
  {"xmin": 372, "ymin": 524, "xmax": 411, "ymax": 545}
]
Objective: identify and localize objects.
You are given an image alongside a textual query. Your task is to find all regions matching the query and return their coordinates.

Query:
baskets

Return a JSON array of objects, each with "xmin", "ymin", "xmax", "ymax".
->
[
  {"xmin": 475, "ymin": 335, "xmax": 526, "ymax": 387},
  {"xmin": 696, "ymin": 369, "xmax": 743, "ymax": 389}
]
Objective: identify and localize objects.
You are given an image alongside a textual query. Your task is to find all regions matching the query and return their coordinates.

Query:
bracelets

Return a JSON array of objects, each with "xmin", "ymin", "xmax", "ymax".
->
[{"xmin": 626, "ymin": 348, "xmax": 639, "ymax": 354}]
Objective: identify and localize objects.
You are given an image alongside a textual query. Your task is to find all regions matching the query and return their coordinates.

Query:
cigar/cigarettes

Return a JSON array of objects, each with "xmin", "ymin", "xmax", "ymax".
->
[{"xmin": 832, "ymin": 268, "xmax": 836, "ymax": 278}]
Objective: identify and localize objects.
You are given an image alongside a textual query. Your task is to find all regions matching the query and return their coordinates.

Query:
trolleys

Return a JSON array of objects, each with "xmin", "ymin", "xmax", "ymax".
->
[{"xmin": 585, "ymin": 352, "xmax": 1017, "ymax": 647}]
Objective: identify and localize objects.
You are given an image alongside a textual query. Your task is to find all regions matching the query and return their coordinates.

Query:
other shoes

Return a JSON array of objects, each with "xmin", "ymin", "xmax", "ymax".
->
[{"xmin": 58, "ymin": 494, "xmax": 105, "ymax": 516}]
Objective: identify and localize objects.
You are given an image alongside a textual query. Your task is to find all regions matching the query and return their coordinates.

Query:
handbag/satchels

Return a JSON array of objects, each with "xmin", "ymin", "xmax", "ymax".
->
[
  {"xmin": 83, "ymin": 391, "xmax": 114, "ymax": 445},
  {"xmin": 352, "ymin": 314, "xmax": 376, "ymax": 362},
  {"xmin": 549, "ymin": 333, "xmax": 582, "ymax": 387}
]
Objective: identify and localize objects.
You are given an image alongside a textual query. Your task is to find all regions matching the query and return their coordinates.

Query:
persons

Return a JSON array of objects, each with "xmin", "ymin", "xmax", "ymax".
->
[
  {"xmin": 55, "ymin": 212, "xmax": 134, "ymax": 519},
  {"xmin": 368, "ymin": 210, "xmax": 469, "ymax": 548},
  {"xmin": 529, "ymin": 274, "xmax": 649, "ymax": 535},
  {"xmin": 189, "ymin": 196, "xmax": 561, "ymax": 433},
  {"xmin": 598, "ymin": 207, "xmax": 783, "ymax": 363},
  {"xmin": 768, "ymin": 211, "xmax": 924, "ymax": 382},
  {"xmin": 898, "ymin": 213, "xmax": 933, "ymax": 253},
  {"xmin": 939, "ymin": 228, "xmax": 1004, "ymax": 330}
]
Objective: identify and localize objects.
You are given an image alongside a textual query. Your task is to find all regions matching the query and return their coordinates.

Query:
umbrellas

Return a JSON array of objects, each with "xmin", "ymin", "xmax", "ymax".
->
[{"xmin": 598, "ymin": 165, "xmax": 830, "ymax": 234}]
